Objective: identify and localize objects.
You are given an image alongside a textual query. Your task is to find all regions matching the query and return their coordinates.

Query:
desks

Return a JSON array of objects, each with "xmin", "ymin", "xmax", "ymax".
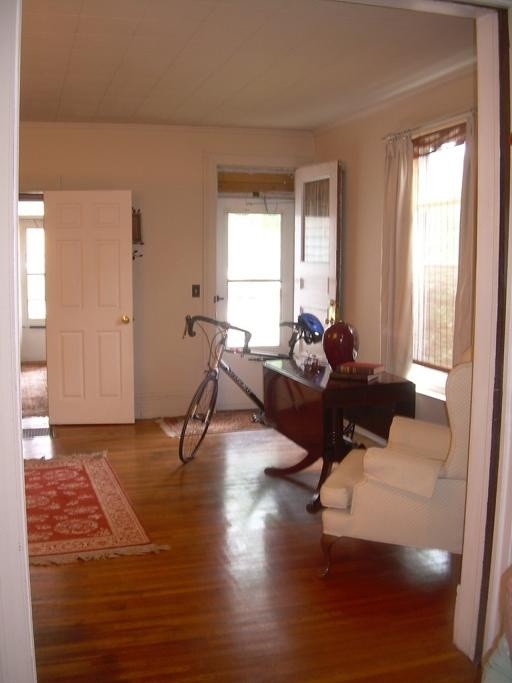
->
[{"xmin": 263, "ymin": 356, "xmax": 416, "ymax": 512}]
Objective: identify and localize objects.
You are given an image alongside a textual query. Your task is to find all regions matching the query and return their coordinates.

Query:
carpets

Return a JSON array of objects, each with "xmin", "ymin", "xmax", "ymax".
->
[
  {"xmin": 23, "ymin": 448, "xmax": 170, "ymax": 567},
  {"xmin": 152, "ymin": 410, "xmax": 272, "ymax": 438}
]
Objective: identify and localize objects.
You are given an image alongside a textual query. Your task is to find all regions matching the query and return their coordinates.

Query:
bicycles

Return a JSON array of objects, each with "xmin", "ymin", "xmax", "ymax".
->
[{"xmin": 178, "ymin": 314, "xmax": 356, "ymax": 464}]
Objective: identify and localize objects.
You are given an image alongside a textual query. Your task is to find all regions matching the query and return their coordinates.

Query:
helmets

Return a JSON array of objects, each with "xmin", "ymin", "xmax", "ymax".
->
[{"xmin": 299, "ymin": 313, "xmax": 324, "ymax": 344}]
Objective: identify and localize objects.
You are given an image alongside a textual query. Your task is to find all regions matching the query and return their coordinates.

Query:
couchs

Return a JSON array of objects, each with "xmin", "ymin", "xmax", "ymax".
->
[{"xmin": 316, "ymin": 363, "xmax": 473, "ymax": 580}]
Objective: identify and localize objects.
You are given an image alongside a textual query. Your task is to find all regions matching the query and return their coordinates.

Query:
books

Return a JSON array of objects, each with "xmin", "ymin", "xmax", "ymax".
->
[
  {"xmin": 329, "ymin": 370, "xmax": 380, "ymax": 382},
  {"xmin": 338, "ymin": 361, "xmax": 386, "ymax": 375}
]
